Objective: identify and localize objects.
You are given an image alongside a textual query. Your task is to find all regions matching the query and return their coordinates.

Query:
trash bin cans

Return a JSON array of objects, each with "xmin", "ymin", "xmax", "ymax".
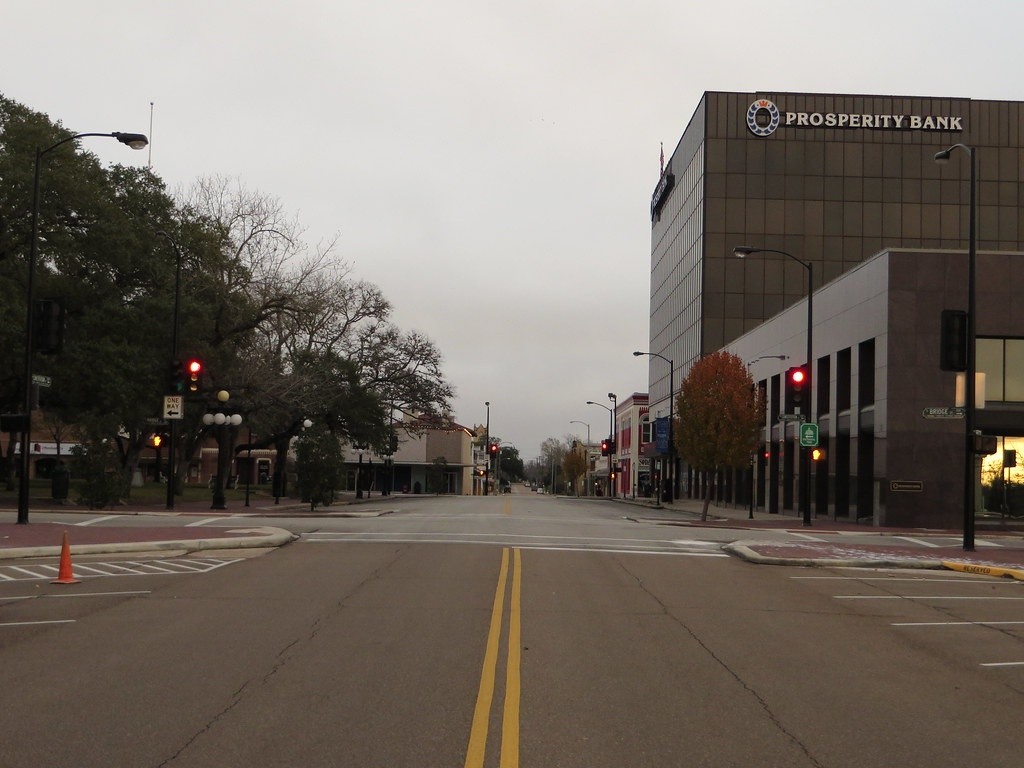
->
[
  {"xmin": 52, "ymin": 469, "xmax": 69, "ymax": 498},
  {"xmin": 414, "ymin": 482, "xmax": 421, "ymax": 494}
]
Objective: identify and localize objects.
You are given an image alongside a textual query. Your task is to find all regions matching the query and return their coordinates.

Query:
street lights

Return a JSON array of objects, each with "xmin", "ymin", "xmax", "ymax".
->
[
  {"xmin": 484, "ymin": 402, "xmax": 490, "ymax": 497},
  {"xmin": 16, "ymin": 133, "xmax": 152, "ymax": 527},
  {"xmin": 350, "ymin": 436, "xmax": 371, "ymax": 500},
  {"xmin": 935, "ymin": 142, "xmax": 975, "ymax": 552},
  {"xmin": 570, "ymin": 420, "xmax": 590, "ymax": 499},
  {"xmin": 201, "ymin": 390, "xmax": 243, "ymax": 513},
  {"xmin": 632, "ymin": 350, "xmax": 676, "ymax": 503},
  {"xmin": 154, "ymin": 231, "xmax": 180, "ymax": 508},
  {"xmin": 381, "ymin": 443, "xmax": 396, "ymax": 498},
  {"xmin": 586, "ymin": 401, "xmax": 613, "ymax": 498},
  {"xmin": 732, "ymin": 244, "xmax": 811, "ymax": 528}
]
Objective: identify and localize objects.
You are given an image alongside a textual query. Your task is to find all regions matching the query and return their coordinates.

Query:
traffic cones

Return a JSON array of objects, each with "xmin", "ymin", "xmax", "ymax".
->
[{"xmin": 48, "ymin": 529, "xmax": 82, "ymax": 584}]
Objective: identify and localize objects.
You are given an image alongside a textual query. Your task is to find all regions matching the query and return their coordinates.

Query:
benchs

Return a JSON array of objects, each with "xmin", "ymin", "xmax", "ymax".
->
[{"xmin": 208, "ymin": 473, "xmax": 239, "ymax": 490}]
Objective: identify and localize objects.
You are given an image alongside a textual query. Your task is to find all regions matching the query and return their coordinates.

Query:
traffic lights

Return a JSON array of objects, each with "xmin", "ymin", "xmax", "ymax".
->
[
  {"xmin": 186, "ymin": 358, "xmax": 202, "ymax": 393},
  {"xmin": 811, "ymin": 448, "xmax": 822, "ymax": 463},
  {"xmin": 490, "ymin": 445, "xmax": 497, "ymax": 458},
  {"xmin": 602, "ymin": 443, "xmax": 609, "ymax": 457},
  {"xmin": 789, "ymin": 366, "xmax": 807, "ymax": 407}
]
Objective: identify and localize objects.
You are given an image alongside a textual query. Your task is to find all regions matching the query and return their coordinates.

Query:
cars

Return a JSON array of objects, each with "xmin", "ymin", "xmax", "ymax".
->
[{"xmin": 524, "ymin": 481, "xmax": 548, "ymax": 493}]
